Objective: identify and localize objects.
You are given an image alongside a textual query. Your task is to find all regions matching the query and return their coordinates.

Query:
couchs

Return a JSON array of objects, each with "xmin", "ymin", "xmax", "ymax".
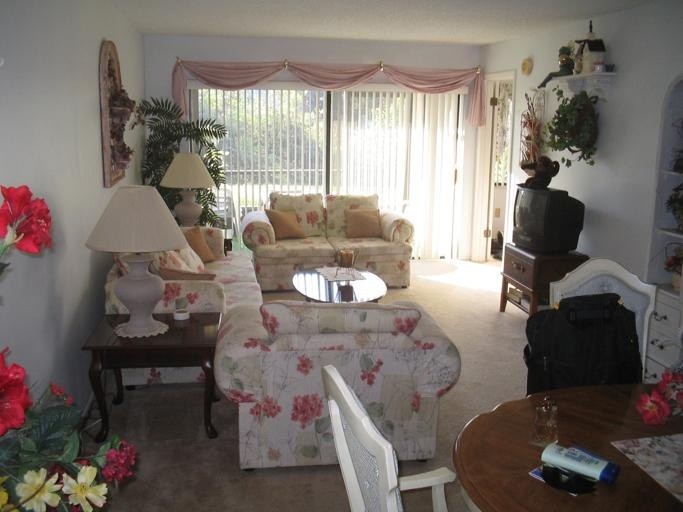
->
[
  {"xmin": 101, "ymin": 228, "xmax": 264, "ymax": 392},
  {"xmin": 238, "ymin": 191, "xmax": 414, "ymax": 292}
]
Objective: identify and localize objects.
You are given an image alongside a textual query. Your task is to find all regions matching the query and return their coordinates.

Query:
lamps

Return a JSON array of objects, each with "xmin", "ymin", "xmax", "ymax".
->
[
  {"xmin": 158, "ymin": 151, "xmax": 216, "ymax": 227},
  {"xmin": 83, "ymin": 183, "xmax": 190, "ymax": 340}
]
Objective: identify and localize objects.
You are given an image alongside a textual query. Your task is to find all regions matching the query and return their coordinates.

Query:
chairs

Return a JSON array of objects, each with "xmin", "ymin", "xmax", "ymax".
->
[
  {"xmin": 547, "ymin": 255, "xmax": 657, "ymax": 383},
  {"xmin": 524, "ymin": 290, "xmax": 643, "ymax": 398},
  {"xmin": 212, "ymin": 300, "xmax": 462, "ymax": 473},
  {"xmin": 316, "ymin": 362, "xmax": 456, "ymax": 512}
]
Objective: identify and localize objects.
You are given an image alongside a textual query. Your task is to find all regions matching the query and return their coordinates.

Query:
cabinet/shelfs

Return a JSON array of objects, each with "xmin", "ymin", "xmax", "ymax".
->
[
  {"xmin": 496, "ymin": 241, "xmax": 590, "ymax": 319},
  {"xmin": 641, "ymin": 76, "xmax": 682, "ymax": 298},
  {"xmin": 644, "ymin": 289, "xmax": 681, "ymax": 386}
]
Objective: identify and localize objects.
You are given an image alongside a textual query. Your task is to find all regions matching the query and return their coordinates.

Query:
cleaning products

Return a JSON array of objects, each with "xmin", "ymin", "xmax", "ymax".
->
[{"xmin": 535, "ymin": 395, "xmax": 560, "ymax": 436}]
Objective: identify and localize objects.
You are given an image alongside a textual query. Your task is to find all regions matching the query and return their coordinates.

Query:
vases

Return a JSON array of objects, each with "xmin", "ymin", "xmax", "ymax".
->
[{"xmin": 670, "ymin": 269, "xmax": 680, "ymax": 292}]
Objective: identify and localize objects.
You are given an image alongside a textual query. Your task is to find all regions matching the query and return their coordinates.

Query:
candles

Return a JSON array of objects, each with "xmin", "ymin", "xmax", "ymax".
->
[{"xmin": 338, "ymin": 249, "xmax": 354, "ymax": 267}]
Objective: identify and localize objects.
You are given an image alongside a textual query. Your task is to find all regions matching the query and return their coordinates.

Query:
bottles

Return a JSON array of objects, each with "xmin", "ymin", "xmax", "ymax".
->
[
  {"xmin": 542, "ymin": 442, "xmax": 622, "ymax": 485},
  {"xmin": 558, "ymin": 46, "xmax": 570, "ymax": 72}
]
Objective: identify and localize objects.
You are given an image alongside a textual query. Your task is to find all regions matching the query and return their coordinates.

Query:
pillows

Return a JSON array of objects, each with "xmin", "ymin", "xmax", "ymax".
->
[
  {"xmin": 340, "ymin": 208, "xmax": 383, "ymax": 239},
  {"xmin": 160, "ymin": 268, "xmax": 214, "ymax": 281},
  {"xmin": 183, "ymin": 225, "xmax": 217, "ymax": 265},
  {"xmin": 264, "ymin": 206, "xmax": 306, "ymax": 241}
]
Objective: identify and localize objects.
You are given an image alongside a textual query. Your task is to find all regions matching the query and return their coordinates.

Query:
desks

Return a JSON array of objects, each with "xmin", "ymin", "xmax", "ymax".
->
[
  {"xmin": 77, "ymin": 310, "xmax": 225, "ymax": 443},
  {"xmin": 450, "ymin": 383, "xmax": 682, "ymax": 512}
]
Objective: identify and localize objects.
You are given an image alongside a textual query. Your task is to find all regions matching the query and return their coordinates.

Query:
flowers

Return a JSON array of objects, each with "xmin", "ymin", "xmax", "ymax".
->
[
  {"xmin": 635, "ymin": 360, "xmax": 682, "ymax": 426},
  {"xmin": 1, "ymin": 182, "xmax": 139, "ymax": 512},
  {"xmin": 662, "ymin": 241, "xmax": 682, "ymax": 277}
]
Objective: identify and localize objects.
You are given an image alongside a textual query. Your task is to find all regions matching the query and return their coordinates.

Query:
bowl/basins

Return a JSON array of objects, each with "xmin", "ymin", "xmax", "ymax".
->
[{"xmin": 172, "ymin": 309, "xmax": 190, "ymax": 320}]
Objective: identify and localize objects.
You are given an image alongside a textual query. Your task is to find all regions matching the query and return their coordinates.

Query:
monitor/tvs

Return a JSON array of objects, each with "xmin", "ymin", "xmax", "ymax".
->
[{"xmin": 512, "ymin": 185, "xmax": 585, "ymax": 253}]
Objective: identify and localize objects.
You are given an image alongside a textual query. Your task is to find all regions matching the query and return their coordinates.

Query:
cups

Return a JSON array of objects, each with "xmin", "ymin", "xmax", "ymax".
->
[
  {"xmin": 606, "ymin": 63, "xmax": 615, "ymax": 72},
  {"xmin": 594, "ymin": 64, "xmax": 604, "ymax": 72}
]
